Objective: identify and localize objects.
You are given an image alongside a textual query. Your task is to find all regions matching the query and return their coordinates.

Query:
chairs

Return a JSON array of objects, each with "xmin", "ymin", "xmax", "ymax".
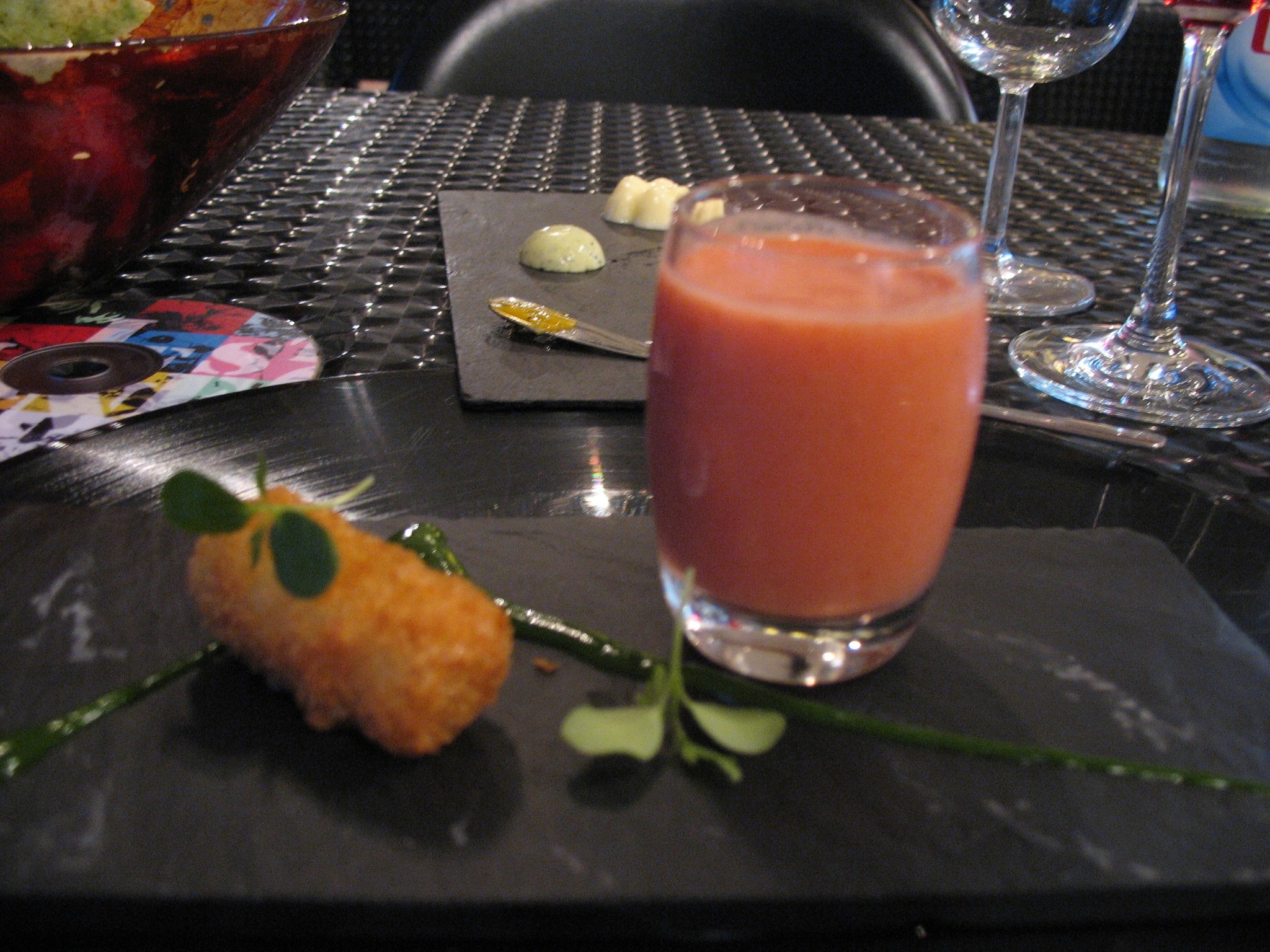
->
[{"xmin": 419, "ymin": 0, "xmax": 985, "ymax": 131}]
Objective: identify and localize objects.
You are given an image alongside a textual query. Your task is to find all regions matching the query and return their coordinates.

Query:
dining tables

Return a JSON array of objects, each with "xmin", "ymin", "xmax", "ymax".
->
[{"xmin": 0, "ymin": 79, "xmax": 1268, "ymax": 951}]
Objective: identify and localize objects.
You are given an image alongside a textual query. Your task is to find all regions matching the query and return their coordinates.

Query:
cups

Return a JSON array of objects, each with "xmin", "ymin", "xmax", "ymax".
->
[{"xmin": 643, "ymin": 161, "xmax": 987, "ymax": 688}]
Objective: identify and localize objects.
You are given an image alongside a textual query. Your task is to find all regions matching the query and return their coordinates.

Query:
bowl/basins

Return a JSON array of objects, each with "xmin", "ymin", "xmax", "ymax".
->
[{"xmin": 0, "ymin": 0, "xmax": 347, "ymax": 315}]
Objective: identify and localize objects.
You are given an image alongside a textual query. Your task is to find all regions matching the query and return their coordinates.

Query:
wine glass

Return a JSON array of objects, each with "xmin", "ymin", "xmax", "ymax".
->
[
  {"xmin": 927, "ymin": 1, "xmax": 1139, "ymax": 315},
  {"xmin": 1007, "ymin": 0, "xmax": 1268, "ymax": 427}
]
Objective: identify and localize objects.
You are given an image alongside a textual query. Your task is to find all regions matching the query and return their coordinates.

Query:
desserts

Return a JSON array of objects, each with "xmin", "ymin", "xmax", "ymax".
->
[
  {"xmin": 519, "ymin": 223, "xmax": 606, "ymax": 272},
  {"xmin": 186, "ymin": 485, "xmax": 517, "ymax": 757},
  {"xmin": 601, "ymin": 174, "xmax": 726, "ymax": 231}
]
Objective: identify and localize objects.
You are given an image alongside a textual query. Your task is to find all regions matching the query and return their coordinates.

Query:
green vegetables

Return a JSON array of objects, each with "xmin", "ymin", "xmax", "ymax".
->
[
  {"xmin": 160, "ymin": 450, "xmax": 377, "ymax": 599},
  {"xmin": 558, "ymin": 566, "xmax": 786, "ymax": 790}
]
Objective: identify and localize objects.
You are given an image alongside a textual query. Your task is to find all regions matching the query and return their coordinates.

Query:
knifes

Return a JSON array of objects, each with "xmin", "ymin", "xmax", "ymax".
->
[{"xmin": 493, "ymin": 294, "xmax": 1167, "ymax": 447}]
